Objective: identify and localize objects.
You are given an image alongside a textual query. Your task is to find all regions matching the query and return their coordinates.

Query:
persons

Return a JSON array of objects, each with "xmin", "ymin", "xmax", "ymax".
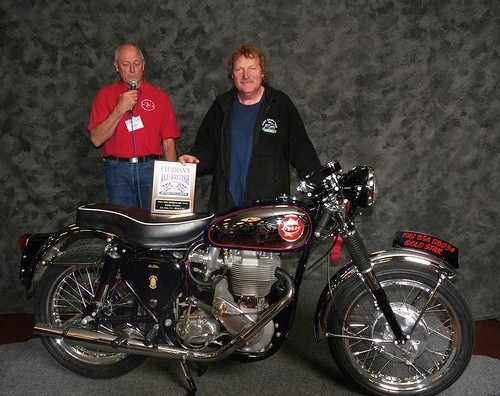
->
[
  {"xmin": 173, "ymin": 43, "xmax": 322, "ymax": 216},
  {"xmin": 88, "ymin": 42, "xmax": 181, "ymax": 210}
]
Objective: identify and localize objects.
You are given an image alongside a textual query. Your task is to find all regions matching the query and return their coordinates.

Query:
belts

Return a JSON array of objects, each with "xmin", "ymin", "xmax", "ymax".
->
[{"xmin": 103, "ymin": 155, "xmax": 163, "ymax": 164}]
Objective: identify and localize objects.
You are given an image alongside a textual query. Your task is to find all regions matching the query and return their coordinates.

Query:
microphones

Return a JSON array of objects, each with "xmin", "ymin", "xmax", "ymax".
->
[{"xmin": 129, "ymin": 79, "xmax": 137, "ymax": 112}]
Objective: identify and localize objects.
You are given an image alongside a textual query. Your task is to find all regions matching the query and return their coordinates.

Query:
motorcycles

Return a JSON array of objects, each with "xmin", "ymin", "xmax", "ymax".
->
[{"xmin": 19, "ymin": 160, "xmax": 477, "ymax": 396}]
